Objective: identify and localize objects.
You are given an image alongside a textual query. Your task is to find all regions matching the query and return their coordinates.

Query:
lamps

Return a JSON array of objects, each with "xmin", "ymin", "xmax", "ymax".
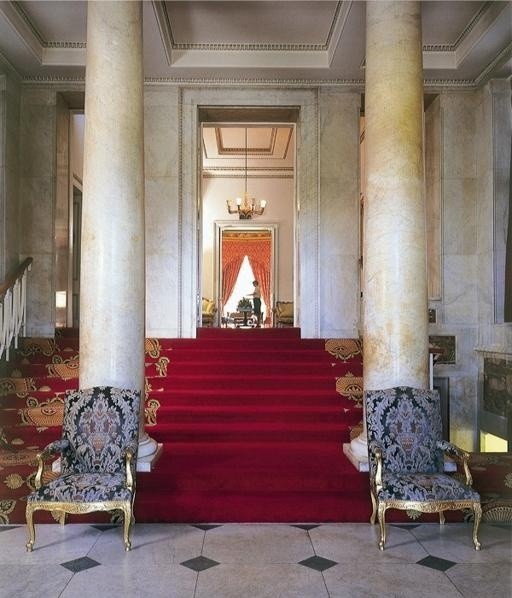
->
[{"xmin": 226, "ymin": 127, "xmax": 266, "ymax": 220}]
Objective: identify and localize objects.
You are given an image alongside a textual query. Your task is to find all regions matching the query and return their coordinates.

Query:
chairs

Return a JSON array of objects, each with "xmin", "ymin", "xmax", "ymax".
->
[
  {"xmin": 24, "ymin": 385, "xmax": 142, "ymax": 554},
  {"xmin": 202, "ymin": 297, "xmax": 218, "ymax": 328},
  {"xmin": 272, "ymin": 301, "xmax": 293, "ymax": 328},
  {"xmin": 364, "ymin": 386, "xmax": 484, "ymax": 554}
]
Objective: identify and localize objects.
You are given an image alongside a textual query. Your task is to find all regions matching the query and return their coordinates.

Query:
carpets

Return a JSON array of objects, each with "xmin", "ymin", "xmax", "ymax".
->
[{"xmin": 0, "ymin": 326, "xmax": 510, "ymax": 525}]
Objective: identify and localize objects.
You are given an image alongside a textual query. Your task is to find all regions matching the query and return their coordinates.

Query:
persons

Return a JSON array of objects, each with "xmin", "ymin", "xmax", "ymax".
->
[{"xmin": 246, "ymin": 280, "xmax": 262, "ymax": 326}]
